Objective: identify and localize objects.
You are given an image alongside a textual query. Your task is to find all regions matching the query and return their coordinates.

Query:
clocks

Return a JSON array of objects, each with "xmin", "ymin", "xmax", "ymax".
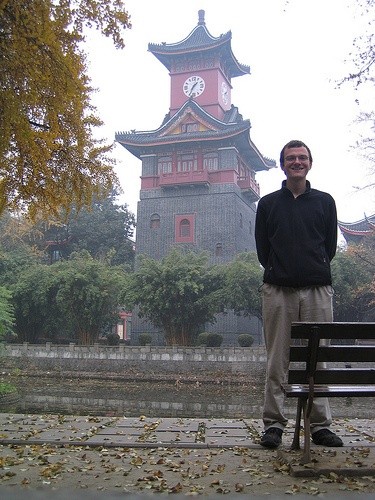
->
[
  {"xmin": 183, "ymin": 76, "xmax": 205, "ymax": 99},
  {"xmin": 221, "ymin": 83, "xmax": 232, "ymax": 104}
]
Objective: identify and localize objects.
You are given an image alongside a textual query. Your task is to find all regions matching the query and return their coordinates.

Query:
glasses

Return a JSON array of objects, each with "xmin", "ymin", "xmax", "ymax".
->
[{"xmin": 283, "ymin": 155, "xmax": 309, "ymax": 161}]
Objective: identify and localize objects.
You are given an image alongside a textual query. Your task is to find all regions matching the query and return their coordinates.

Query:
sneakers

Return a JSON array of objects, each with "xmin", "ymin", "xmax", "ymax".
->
[
  {"xmin": 260, "ymin": 428, "xmax": 282, "ymax": 449},
  {"xmin": 312, "ymin": 429, "xmax": 343, "ymax": 447}
]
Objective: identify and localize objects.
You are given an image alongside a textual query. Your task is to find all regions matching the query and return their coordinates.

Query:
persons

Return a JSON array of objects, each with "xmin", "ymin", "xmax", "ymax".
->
[{"xmin": 255, "ymin": 140, "xmax": 344, "ymax": 449}]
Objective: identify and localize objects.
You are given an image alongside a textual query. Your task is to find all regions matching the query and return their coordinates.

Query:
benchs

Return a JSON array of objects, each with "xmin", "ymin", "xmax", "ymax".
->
[{"xmin": 280, "ymin": 322, "xmax": 375, "ymax": 466}]
